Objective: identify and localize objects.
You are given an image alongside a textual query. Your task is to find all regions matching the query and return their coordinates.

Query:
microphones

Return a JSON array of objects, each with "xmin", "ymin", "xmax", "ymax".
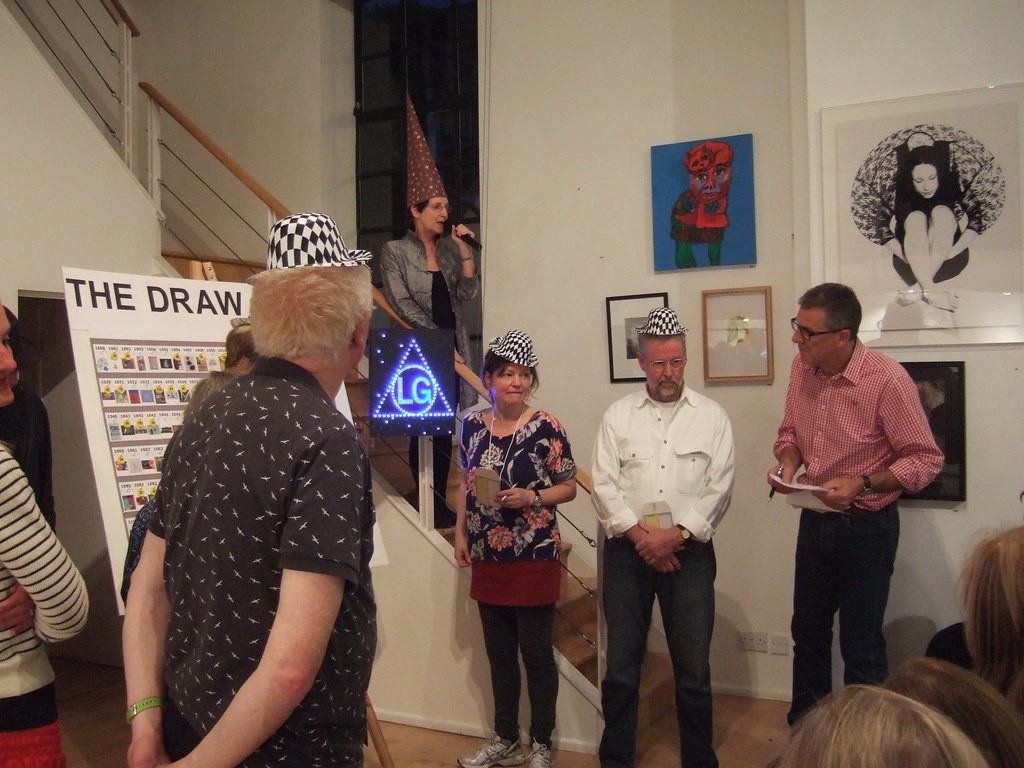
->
[{"xmin": 448, "ymin": 225, "xmax": 482, "ymax": 251}]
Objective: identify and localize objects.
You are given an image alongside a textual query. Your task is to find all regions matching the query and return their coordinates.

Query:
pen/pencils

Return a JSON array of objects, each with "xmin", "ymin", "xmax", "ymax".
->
[
  {"xmin": 501, "ymin": 482, "xmax": 520, "ymax": 501},
  {"xmin": 767, "ymin": 467, "xmax": 784, "ymax": 503}
]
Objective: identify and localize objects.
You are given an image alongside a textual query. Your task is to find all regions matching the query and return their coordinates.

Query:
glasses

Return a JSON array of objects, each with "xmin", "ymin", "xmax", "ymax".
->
[
  {"xmin": 790, "ymin": 317, "xmax": 850, "ymax": 341},
  {"xmin": 639, "ymin": 353, "xmax": 687, "ymax": 369}
]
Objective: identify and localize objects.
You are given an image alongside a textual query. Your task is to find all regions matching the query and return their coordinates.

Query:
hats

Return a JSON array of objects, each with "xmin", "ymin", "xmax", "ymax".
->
[
  {"xmin": 406, "ymin": 90, "xmax": 446, "ymax": 207},
  {"xmin": 489, "ymin": 328, "xmax": 539, "ymax": 367},
  {"xmin": 634, "ymin": 308, "xmax": 689, "ymax": 336},
  {"xmin": 246, "ymin": 211, "xmax": 372, "ymax": 284}
]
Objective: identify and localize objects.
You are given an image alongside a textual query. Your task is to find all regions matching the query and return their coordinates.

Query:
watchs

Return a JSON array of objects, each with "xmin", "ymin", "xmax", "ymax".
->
[
  {"xmin": 533, "ymin": 489, "xmax": 541, "ymax": 506},
  {"xmin": 676, "ymin": 524, "xmax": 690, "ymax": 540},
  {"xmin": 862, "ymin": 476, "xmax": 872, "ymax": 496}
]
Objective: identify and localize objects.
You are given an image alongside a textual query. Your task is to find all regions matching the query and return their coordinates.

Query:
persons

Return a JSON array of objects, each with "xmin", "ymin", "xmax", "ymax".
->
[
  {"xmin": 767, "ymin": 283, "xmax": 944, "ymax": 725},
  {"xmin": 887, "ymin": 658, "xmax": 1024, "ymax": 768},
  {"xmin": 381, "ymin": 195, "xmax": 479, "ymax": 522},
  {"xmin": 0, "ymin": 305, "xmax": 88, "ymax": 767},
  {"xmin": 879, "ymin": 146, "xmax": 982, "ymax": 312},
  {"xmin": 453, "ymin": 328, "xmax": 577, "ymax": 767},
  {"xmin": 591, "ymin": 308, "xmax": 735, "ymax": 768},
  {"xmin": 781, "ymin": 684, "xmax": 985, "ymax": 768},
  {"xmin": 961, "ymin": 526, "xmax": 1024, "ymax": 713},
  {"xmin": 120, "ymin": 214, "xmax": 375, "ymax": 768}
]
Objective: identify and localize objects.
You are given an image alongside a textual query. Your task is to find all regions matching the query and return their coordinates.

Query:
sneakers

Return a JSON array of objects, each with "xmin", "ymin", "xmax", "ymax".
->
[
  {"xmin": 526, "ymin": 739, "xmax": 553, "ymax": 768},
  {"xmin": 457, "ymin": 730, "xmax": 526, "ymax": 768}
]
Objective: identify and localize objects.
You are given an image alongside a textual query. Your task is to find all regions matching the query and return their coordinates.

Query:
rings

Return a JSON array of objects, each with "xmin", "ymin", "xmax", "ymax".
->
[{"xmin": 644, "ymin": 557, "xmax": 649, "ymax": 561}]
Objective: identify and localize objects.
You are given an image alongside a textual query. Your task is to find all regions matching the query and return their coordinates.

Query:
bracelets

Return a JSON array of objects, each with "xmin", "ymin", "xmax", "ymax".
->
[
  {"xmin": 126, "ymin": 697, "xmax": 166, "ymax": 724},
  {"xmin": 463, "ymin": 257, "xmax": 472, "ymax": 261}
]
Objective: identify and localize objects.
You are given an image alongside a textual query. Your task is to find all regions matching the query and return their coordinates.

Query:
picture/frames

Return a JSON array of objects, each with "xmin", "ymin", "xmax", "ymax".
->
[
  {"xmin": 818, "ymin": 82, "xmax": 1024, "ymax": 344},
  {"xmin": 605, "ymin": 292, "xmax": 669, "ymax": 384},
  {"xmin": 894, "ymin": 361, "xmax": 967, "ymax": 501},
  {"xmin": 700, "ymin": 285, "xmax": 775, "ymax": 388}
]
